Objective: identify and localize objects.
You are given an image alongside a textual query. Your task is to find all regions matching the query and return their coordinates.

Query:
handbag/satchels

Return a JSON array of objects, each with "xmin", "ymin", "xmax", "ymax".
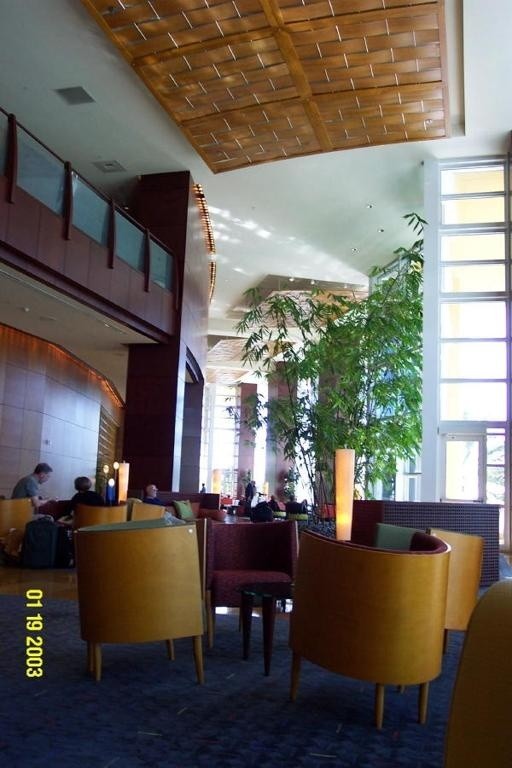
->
[{"xmin": 250, "ymin": 500, "xmax": 274, "ymax": 522}]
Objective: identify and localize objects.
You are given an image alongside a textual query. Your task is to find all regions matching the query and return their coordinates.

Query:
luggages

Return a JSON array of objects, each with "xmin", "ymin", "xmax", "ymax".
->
[{"xmin": 23, "ymin": 516, "xmax": 59, "ymax": 568}]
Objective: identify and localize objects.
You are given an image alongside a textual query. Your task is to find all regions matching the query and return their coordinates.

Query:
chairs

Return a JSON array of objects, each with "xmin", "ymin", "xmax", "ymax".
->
[
  {"xmin": 443, "ymin": 577, "xmax": 512, "ymax": 767},
  {"xmin": 1, "ymin": 495, "xmax": 166, "ymax": 568}
]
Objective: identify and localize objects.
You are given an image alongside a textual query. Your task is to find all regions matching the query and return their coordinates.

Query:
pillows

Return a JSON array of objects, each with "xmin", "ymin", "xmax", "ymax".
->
[{"xmin": 172, "ymin": 498, "xmax": 200, "ymax": 519}]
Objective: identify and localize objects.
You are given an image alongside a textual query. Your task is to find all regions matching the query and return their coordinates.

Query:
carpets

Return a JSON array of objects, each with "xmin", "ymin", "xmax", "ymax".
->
[{"xmin": 0, "ymin": 591, "xmax": 468, "ymax": 767}]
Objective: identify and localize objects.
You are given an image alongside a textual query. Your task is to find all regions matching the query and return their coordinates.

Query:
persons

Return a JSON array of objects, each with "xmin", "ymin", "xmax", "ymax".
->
[
  {"xmin": 200, "ymin": 483, "xmax": 206, "ymax": 493},
  {"xmin": 221, "ymin": 480, "xmax": 308, "ymax": 524},
  {"xmin": 56, "ymin": 476, "xmax": 94, "ymax": 526},
  {"xmin": 10, "ymin": 463, "xmax": 56, "ymax": 510},
  {"xmin": 143, "ymin": 484, "xmax": 165, "ymax": 506}
]
